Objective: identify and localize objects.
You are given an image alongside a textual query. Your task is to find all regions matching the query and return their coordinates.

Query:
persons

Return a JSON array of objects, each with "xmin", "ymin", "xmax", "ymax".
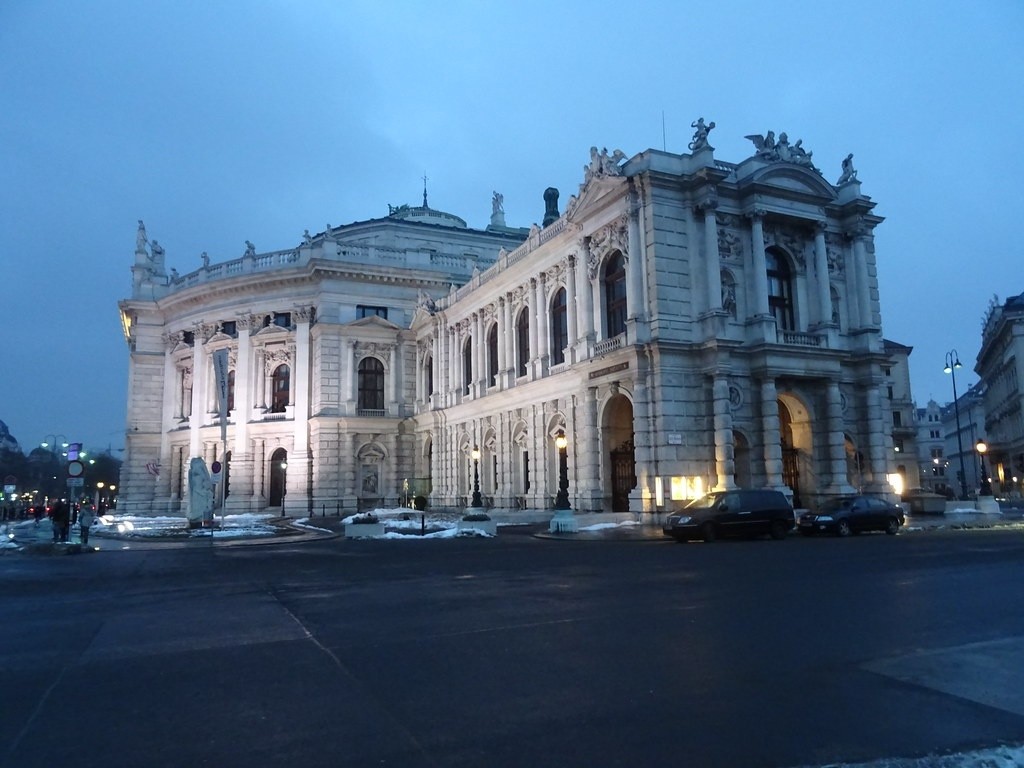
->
[{"xmin": 30, "ymin": 494, "xmax": 117, "ymax": 545}]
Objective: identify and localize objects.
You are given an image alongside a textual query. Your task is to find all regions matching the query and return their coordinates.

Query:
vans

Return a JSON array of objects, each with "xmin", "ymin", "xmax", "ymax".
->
[{"xmin": 664, "ymin": 490, "xmax": 795, "ymax": 542}]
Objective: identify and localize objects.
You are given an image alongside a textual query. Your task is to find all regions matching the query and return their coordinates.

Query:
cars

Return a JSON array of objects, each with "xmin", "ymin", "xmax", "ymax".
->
[{"xmin": 796, "ymin": 493, "xmax": 905, "ymax": 536}]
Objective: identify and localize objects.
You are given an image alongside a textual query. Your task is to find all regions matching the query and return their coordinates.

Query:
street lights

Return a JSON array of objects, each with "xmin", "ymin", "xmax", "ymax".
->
[
  {"xmin": 921, "ymin": 459, "xmax": 949, "ymax": 495},
  {"xmin": 976, "ymin": 438, "xmax": 993, "ymax": 496},
  {"xmin": 0, "ymin": 433, "xmax": 120, "ymax": 524},
  {"xmin": 464, "ymin": 445, "xmax": 491, "ymax": 522},
  {"xmin": 943, "ymin": 349, "xmax": 972, "ymax": 502},
  {"xmin": 279, "ymin": 458, "xmax": 289, "ymax": 517},
  {"xmin": 550, "ymin": 429, "xmax": 578, "ymax": 534}
]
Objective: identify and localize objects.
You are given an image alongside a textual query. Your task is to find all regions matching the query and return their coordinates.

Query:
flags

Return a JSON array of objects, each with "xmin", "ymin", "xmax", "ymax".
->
[{"xmin": 212, "ymin": 349, "xmax": 227, "ymax": 440}]
